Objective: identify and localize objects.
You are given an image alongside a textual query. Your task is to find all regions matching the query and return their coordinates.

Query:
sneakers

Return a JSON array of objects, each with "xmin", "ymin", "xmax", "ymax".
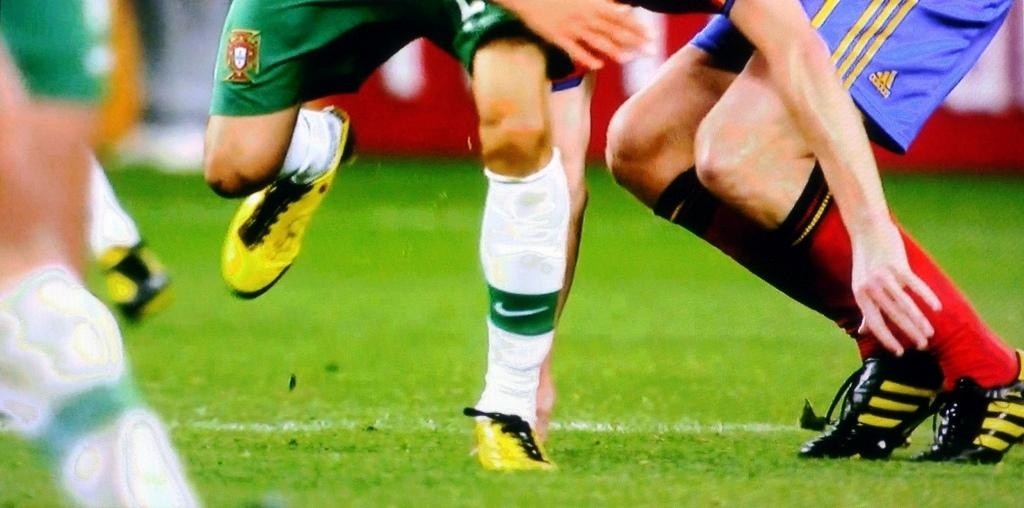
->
[
  {"xmin": 463, "ymin": 407, "xmax": 560, "ymax": 472},
  {"xmin": 798, "ymin": 348, "xmax": 1024, "ymax": 465},
  {"xmin": 99, "ymin": 239, "xmax": 171, "ymax": 324},
  {"xmin": 220, "ymin": 104, "xmax": 359, "ymax": 300}
]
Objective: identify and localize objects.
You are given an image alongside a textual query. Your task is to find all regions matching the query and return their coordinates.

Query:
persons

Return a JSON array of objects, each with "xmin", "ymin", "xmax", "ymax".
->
[
  {"xmin": 0, "ymin": 3, "xmax": 201, "ymax": 506},
  {"xmin": 201, "ymin": 0, "xmax": 656, "ymax": 472},
  {"xmin": 534, "ymin": 0, "xmax": 1024, "ymax": 469}
]
